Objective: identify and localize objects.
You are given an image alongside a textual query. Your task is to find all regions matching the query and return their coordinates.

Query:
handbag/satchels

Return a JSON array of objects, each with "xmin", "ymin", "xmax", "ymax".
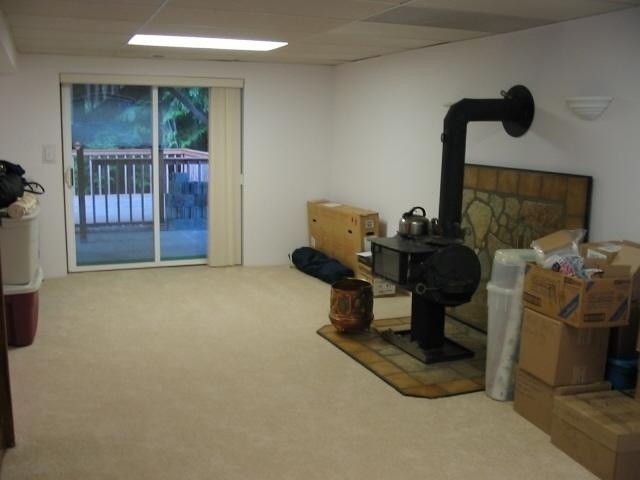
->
[{"xmin": 0, "ymin": 173, "xmax": 45, "ymax": 209}]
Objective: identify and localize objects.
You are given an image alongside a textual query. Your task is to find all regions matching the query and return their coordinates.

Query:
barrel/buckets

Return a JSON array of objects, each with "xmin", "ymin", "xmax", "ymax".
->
[{"xmin": 609, "ymin": 355, "xmax": 637, "ymax": 388}]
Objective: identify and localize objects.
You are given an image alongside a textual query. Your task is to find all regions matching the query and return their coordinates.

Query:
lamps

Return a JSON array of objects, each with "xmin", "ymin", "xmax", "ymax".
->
[{"xmin": 565, "ymin": 95, "xmax": 613, "ymax": 121}]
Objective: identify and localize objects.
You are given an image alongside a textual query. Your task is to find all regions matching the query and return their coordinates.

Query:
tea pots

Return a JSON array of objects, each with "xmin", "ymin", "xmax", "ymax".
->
[{"xmin": 399, "ymin": 206, "xmax": 429, "ymax": 237}]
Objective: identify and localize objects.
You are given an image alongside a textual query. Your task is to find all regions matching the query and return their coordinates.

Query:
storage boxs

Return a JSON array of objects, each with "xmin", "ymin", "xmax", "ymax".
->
[
  {"xmin": 1, "ymin": 203, "xmax": 43, "ymax": 346},
  {"xmin": 305, "ymin": 200, "xmax": 398, "ymax": 299},
  {"xmin": 513, "ymin": 227, "xmax": 640, "ymax": 480}
]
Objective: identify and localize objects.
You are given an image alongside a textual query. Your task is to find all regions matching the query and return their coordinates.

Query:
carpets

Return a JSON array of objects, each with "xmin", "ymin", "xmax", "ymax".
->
[{"xmin": 317, "ymin": 312, "xmax": 487, "ymax": 399}]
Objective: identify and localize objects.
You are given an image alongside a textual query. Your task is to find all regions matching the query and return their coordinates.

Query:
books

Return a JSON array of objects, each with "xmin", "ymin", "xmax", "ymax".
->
[{"xmin": 356, "ymin": 250, "xmax": 397, "ymax": 298}]
